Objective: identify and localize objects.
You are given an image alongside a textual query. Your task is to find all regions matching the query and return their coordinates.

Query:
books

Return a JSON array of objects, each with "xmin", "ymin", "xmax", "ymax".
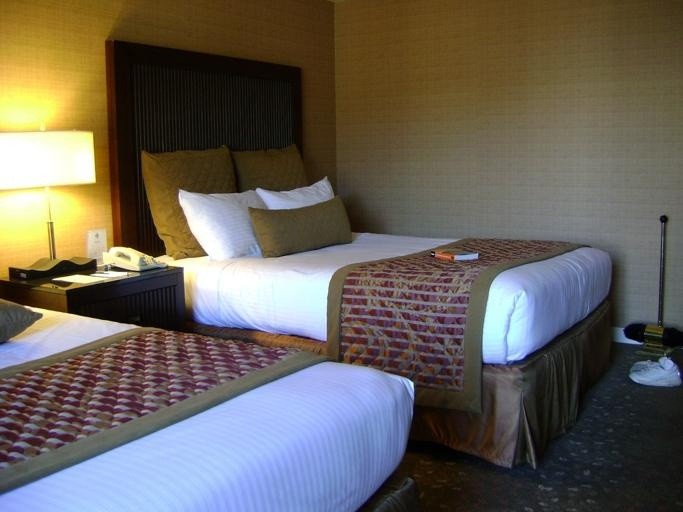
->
[{"xmin": 431, "ymin": 248, "xmax": 478, "ymax": 262}]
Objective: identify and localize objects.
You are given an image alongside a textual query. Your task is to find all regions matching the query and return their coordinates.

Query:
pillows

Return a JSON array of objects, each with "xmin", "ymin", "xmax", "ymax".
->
[
  {"xmin": 255, "ymin": 172, "xmax": 334, "ymax": 212},
  {"xmin": 0, "ymin": 296, "xmax": 42, "ymax": 346},
  {"xmin": 230, "ymin": 144, "xmax": 308, "ymax": 192},
  {"xmin": 247, "ymin": 193, "xmax": 351, "ymax": 260},
  {"xmin": 178, "ymin": 183, "xmax": 270, "ymax": 261},
  {"xmin": 138, "ymin": 140, "xmax": 237, "ymax": 260}
]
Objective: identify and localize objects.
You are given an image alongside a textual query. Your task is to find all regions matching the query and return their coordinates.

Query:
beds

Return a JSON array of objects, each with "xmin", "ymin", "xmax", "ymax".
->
[
  {"xmin": 105, "ymin": 39, "xmax": 617, "ymax": 478},
  {"xmin": 0, "ymin": 299, "xmax": 422, "ymax": 512}
]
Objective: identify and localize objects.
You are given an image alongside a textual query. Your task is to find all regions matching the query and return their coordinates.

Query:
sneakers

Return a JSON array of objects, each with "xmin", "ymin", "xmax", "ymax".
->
[{"xmin": 630, "ymin": 355, "xmax": 681, "ymax": 388}]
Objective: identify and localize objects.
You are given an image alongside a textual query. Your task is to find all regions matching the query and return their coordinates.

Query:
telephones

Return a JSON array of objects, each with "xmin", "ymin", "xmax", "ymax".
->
[{"xmin": 103, "ymin": 246, "xmax": 168, "ymax": 271}]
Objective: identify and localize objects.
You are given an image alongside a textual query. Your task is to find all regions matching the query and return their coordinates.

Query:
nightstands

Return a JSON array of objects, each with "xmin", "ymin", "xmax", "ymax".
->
[{"xmin": 0, "ymin": 263, "xmax": 187, "ymax": 331}]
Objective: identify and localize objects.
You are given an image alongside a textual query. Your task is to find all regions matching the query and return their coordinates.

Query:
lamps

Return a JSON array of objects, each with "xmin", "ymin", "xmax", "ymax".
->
[{"xmin": 0, "ymin": 124, "xmax": 96, "ymax": 280}]
